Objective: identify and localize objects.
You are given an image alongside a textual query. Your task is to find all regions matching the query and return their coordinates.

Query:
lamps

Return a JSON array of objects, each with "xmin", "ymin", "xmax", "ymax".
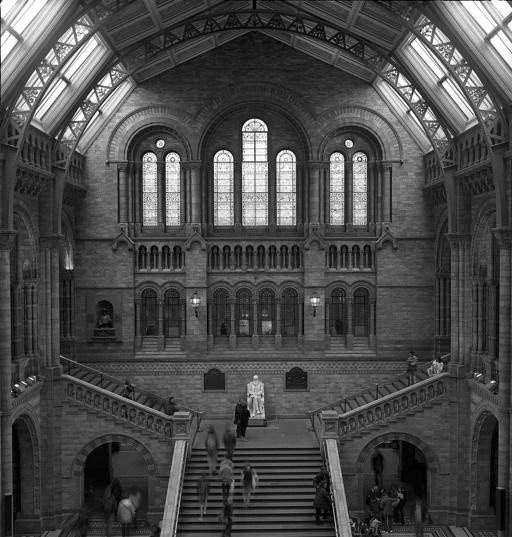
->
[
  {"xmin": 308, "ymin": 290, "xmax": 322, "ymax": 316},
  {"xmin": 188, "ymin": 291, "xmax": 201, "ymax": 317}
]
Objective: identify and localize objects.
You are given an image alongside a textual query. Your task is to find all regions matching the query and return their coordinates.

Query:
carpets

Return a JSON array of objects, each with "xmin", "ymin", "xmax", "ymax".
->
[{"xmin": 379, "ymin": 523, "xmax": 456, "ymax": 537}]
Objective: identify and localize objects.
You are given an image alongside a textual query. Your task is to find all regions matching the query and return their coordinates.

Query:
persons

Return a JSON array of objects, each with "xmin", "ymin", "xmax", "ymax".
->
[
  {"xmin": 313, "ymin": 468, "xmax": 330, "ymax": 492},
  {"xmin": 350, "ymin": 449, "xmax": 408, "ymax": 537},
  {"xmin": 247, "ymin": 375, "xmax": 264, "ymax": 418},
  {"xmin": 98, "ymin": 309, "xmax": 112, "ymax": 328},
  {"xmin": 407, "ymin": 352, "xmax": 417, "ymax": 386},
  {"xmin": 123, "ymin": 381, "xmax": 134, "ymax": 401},
  {"xmin": 75, "ymin": 478, "xmax": 140, "ymax": 537},
  {"xmin": 195, "ymin": 399, "xmax": 258, "ymax": 537},
  {"xmin": 165, "ymin": 397, "xmax": 178, "ymax": 416},
  {"xmin": 313, "ymin": 481, "xmax": 334, "ymax": 527},
  {"xmin": 427, "ymin": 357, "xmax": 444, "ymax": 378}
]
